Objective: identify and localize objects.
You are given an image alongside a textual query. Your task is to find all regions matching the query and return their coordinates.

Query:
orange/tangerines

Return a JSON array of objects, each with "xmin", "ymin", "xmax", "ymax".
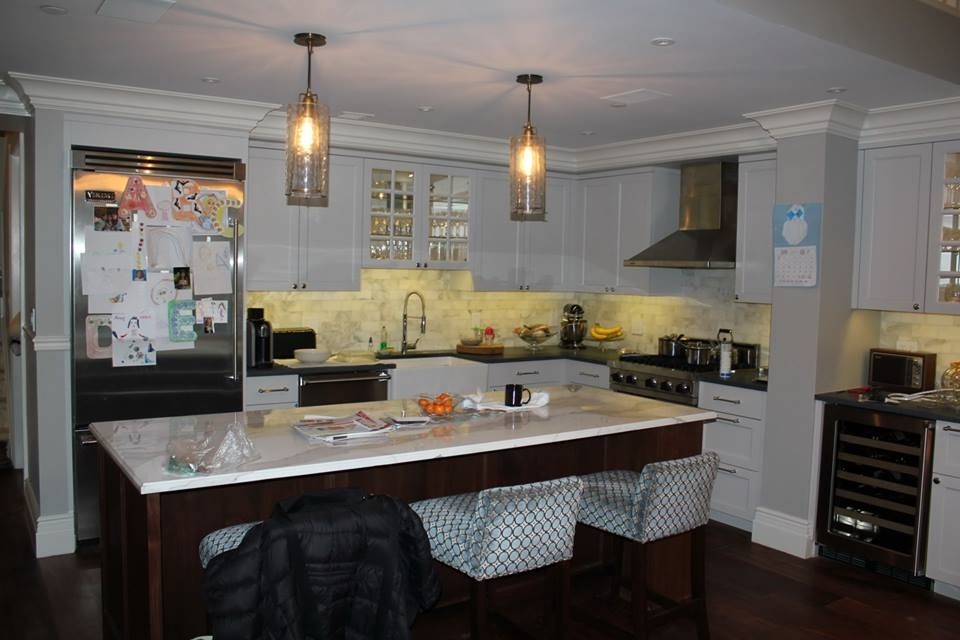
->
[{"xmin": 419, "ymin": 393, "xmax": 454, "ymax": 415}]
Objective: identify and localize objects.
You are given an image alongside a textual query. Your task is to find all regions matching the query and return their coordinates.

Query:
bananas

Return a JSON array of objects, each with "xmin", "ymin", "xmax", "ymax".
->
[{"xmin": 589, "ymin": 322, "xmax": 623, "ymax": 340}]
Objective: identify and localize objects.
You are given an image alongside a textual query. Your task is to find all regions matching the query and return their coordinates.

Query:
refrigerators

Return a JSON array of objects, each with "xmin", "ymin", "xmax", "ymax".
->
[{"xmin": 71, "ymin": 145, "xmax": 243, "ymax": 546}]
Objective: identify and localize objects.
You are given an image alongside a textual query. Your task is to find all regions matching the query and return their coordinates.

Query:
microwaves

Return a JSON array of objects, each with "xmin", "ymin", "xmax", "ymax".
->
[{"xmin": 868, "ymin": 347, "xmax": 937, "ymax": 395}]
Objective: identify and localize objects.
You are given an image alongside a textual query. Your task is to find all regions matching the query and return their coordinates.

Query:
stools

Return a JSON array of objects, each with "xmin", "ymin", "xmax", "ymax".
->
[
  {"xmin": 409, "ymin": 474, "xmax": 582, "ymax": 640},
  {"xmin": 197, "ymin": 487, "xmax": 441, "ymax": 640},
  {"xmin": 575, "ymin": 451, "xmax": 722, "ymax": 640}
]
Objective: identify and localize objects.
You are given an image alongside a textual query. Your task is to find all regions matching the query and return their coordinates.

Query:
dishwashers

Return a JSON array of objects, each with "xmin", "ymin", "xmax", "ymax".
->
[{"xmin": 299, "ymin": 374, "xmax": 391, "ymax": 407}]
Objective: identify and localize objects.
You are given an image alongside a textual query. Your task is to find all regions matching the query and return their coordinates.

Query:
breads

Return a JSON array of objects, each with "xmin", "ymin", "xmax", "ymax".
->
[{"xmin": 514, "ymin": 324, "xmax": 550, "ymax": 343}]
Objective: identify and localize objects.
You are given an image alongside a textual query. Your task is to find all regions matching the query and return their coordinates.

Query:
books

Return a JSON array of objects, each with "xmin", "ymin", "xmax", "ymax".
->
[{"xmin": 293, "ymin": 411, "xmax": 393, "ymax": 442}]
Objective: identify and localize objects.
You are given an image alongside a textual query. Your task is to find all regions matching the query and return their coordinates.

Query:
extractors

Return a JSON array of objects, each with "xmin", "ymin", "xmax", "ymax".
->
[{"xmin": 622, "ymin": 160, "xmax": 737, "ymax": 270}]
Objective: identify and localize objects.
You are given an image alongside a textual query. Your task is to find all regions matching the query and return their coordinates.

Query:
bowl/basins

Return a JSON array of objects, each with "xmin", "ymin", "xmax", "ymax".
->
[
  {"xmin": 379, "ymin": 350, "xmax": 391, "ymax": 355},
  {"xmin": 294, "ymin": 349, "xmax": 332, "ymax": 363},
  {"xmin": 560, "ymin": 323, "xmax": 587, "ymax": 343},
  {"xmin": 461, "ymin": 338, "xmax": 482, "ymax": 346},
  {"xmin": 412, "ymin": 392, "xmax": 464, "ymax": 420}
]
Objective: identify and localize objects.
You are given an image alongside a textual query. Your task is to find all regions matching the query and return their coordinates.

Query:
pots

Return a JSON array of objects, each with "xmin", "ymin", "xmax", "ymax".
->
[
  {"xmin": 667, "ymin": 336, "xmax": 711, "ymax": 365},
  {"xmin": 658, "ymin": 333, "xmax": 687, "ymax": 357}
]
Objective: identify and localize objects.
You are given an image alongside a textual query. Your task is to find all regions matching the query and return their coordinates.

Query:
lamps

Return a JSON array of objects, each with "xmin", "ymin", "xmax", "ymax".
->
[
  {"xmin": 283, "ymin": 33, "xmax": 330, "ymax": 198},
  {"xmin": 509, "ymin": 74, "xmax": 546, "ymax": 212}
]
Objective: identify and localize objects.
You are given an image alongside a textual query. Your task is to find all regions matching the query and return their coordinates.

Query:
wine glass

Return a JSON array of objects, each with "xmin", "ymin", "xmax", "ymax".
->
[
  {"xmin": 370, "ymin": 207, "xmax": 467, "ymax": 238},
  {"xmin": 943, "ymin": 175, "xmax": 960, "ymax": 207}
]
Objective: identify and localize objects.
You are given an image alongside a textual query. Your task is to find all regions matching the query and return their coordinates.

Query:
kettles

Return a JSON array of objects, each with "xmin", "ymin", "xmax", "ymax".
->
[{"xmin": 710, "ymin": 328, "xmax": 737, "ymax": 365}]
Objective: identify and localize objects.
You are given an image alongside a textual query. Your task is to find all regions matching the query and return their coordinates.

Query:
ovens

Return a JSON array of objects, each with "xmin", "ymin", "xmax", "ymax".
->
[{"xmin": 610, "ymin": 369, "xmax": 699, "ymax": 408}]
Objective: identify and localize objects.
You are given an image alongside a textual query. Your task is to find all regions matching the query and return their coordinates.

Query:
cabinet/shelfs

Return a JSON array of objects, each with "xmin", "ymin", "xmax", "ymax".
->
[
  {"xmin": 470, "ymin": 169, "xmax": 569, "ymax": 293},
  {"xmin": 362, "ymin": 157, "xmax": 472, "ymax": 270},
  {"xmin": 855, "ymin": 143, "xmax": 930, "ymax": 311},
  {"xmin": 925, "ymin": 138, "xmax": 960, "ymax": 315},
  {"xmin": 245, "ymin": 139, "xmax": 362, "ymax": 291},
  {"xmin": 488, "ymin": 360, "xmax": 566, "ymax": 392},
  {"xmin": 924, "ymin": 422, "xmax": 960, "ymax": 587},
  {"xmin": 697, "ymin": 381, "xmax": 766, "ymax": 519},
  {"xmin": 246, "ymin": 376, "xmax": 297, "ymax": 411},
  {"xmin": 570, "ymin": 173, "xmax": 652, "ymax": 292},
  {"xmin": 733, "ymin": 150, "xmax": 777, "ymax": 302},
  {"xmin": 566, "ymin": 359, "xmax": 609, "ymax": 387}
]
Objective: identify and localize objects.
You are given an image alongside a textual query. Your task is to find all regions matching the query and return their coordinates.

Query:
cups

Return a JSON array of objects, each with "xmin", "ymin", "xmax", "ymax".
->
[
  {"xmin": 504, "ymin": 384, "xmax": 531, "ymax": 407},
  {"xmin": 371, "ymin": 240, "xmax": 411, "ymax": 260},
  {"xmin": 429, "ymin": 241, "xmax": 467, "ymax": 260}
]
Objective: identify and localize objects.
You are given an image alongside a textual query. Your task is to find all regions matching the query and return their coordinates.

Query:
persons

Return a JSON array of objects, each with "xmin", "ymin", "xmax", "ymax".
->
[
  {"xmin": 178, "ymin": 272, "xmax": 188, "ymax": 285},
  {"xmin": 95, "ymin": 213, "xmax": 124, "ymax": 231},
  {"xmin": 134, "ymin": 270, "xmax": 144, "ymax": 280},
  {"xmin": 204, "ymin": 319, "xmax": 213, "ymax": 333}
]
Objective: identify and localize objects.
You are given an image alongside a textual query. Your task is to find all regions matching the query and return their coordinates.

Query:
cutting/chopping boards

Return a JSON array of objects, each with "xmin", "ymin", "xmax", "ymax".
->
[{"xmin": 456, "ymin": 343, "xmax": 504, "ymax": 355}]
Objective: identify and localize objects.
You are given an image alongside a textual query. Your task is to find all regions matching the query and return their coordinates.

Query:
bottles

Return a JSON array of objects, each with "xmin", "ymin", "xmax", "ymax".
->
[
  {"xmin": 941, "ymin": 362, "xmax": 960, "ymax": 401},
  {"xmin": 380, "ymin": 326, "xmax": 388, "ymax": 350}
]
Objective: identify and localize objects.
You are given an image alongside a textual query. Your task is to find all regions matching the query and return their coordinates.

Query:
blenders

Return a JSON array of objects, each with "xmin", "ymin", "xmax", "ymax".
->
[{"xmin": 560, "ymin": 303, "xmax": 586, "ymax": 348}]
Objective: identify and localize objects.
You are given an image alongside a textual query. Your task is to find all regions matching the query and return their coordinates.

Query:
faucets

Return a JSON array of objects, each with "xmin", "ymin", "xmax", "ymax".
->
[{"xmin": 401, "ymin": 291, "xmax": 427, "ymax": 356}]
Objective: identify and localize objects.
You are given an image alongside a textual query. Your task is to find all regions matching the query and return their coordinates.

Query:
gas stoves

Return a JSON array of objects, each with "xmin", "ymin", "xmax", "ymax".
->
[{"xmin": 617, "ymin": 335, "xmax": 760, "ymax": 380}]
